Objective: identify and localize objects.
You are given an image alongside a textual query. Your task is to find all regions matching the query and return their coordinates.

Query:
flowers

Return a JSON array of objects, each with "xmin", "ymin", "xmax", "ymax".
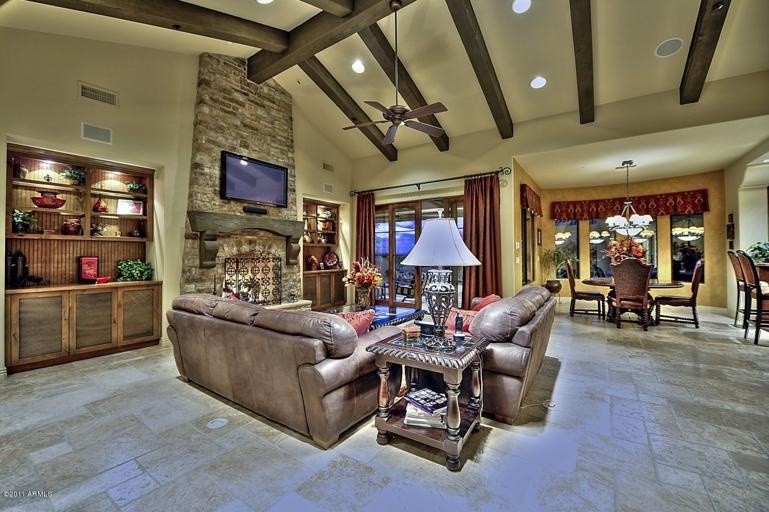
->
[
  {"xmin": 600, "ymin": 236, "xmax": 648, "ymax": 263},
  {"xmin": 340, "ymin": 256, "xmax": 386, "ymax": 290}
]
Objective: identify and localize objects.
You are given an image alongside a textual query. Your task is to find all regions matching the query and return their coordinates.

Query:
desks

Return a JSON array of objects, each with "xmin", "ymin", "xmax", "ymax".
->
[
  {"xmin": 578, "ymin": 277, "xmax": 685, "ymax": 325},
  {"xmin": 365, "ymin": 330, "xmax": 497, "ymax": 474},
  {"xmin": 751, "ymin": 261, "xmax": 769, "ymax": 320}
]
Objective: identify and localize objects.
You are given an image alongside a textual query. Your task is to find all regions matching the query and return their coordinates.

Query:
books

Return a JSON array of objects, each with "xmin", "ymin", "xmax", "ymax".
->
[{"xmin": 402, "ymin": 387, "xmax": 448, "ymax": 430}]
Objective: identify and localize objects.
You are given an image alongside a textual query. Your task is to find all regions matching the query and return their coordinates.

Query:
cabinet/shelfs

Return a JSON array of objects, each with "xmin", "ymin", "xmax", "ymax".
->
[
  {"xmin": 301, "ymin": 196, "xmax": 339, "ymax": 246},
  {"xmin": 3, "ymin": 141, "xmax": 157, "ymax": 245},
  {"xmin": 4, "ymin": 273, "xmax": 167, "ymax": 379},
  {"xmin": 303, "ymin": 269, "xmax": 349, "ymax": 311}
]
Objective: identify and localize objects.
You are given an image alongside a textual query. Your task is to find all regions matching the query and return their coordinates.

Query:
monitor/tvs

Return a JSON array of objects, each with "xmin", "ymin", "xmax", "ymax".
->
[{"xmin": 220, "ymin": 151, "xmax": 288, "ymax": 209}]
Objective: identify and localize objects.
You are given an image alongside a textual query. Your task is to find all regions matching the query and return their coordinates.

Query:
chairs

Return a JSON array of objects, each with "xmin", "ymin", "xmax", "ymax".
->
[
  {"xmin": 564, "ymin": 258, "xmax": 607, "ymax": 320},
  {"xmin": 654, "ymin": 258, "xmax": 706, "ymax": 329},
  {"xmin": 607, "ymin": 256, "xmax": 656, "ymax": 332},
  {"xmin": 735, "ymin": 248, "xmax": 769, "ymax": 345},
  {"xmin": 726, "ymin": 249, "xmax": 769, "ymax": 329}
]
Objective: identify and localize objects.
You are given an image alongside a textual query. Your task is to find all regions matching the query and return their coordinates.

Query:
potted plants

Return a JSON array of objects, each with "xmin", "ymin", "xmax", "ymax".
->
[
  {"xmin": 124, "ymin": 178, "xmax": 146, "ymax": 196},
  {"xmin": 59, "ymin": 165, "xmax": 84, "ymax": 188},
  {"xmin": 542, "ymin": 245, "xmax": 582, "ymax": 293}
]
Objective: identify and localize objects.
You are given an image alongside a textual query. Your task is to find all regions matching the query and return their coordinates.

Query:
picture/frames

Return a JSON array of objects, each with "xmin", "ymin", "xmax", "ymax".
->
[
  {"xmin": 116, "ymin": 198, "xmax": 146, "ymax": 217},
  {"xmin": 537, "ymin": 228, "xmax": 543, "ymax": 245}
]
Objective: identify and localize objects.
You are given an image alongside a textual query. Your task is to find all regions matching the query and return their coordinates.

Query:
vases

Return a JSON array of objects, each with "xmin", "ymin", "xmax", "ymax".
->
[
  {"xmin": 28, "ymin": 188, "xmax": 69, "ymax": 209},
  {"xmin": 353, "ymin": 285, "xmax": 374, "ymax": 311},
  {"xmin": 92, "ymin": 191, "xmax": 112, "ymax": 213},
  {"xmin": 61, "ymin": 218, "xmax": 82, "ymax": 237},
  {"xmin": 14, "ymin": 163, "xmax": 29, "ymax": 180}
]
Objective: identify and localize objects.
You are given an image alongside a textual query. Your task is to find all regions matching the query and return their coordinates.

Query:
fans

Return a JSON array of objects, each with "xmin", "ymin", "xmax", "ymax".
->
[{"xmin": 338, "ymin": 0, "xmax": 453, "ymax": 148}]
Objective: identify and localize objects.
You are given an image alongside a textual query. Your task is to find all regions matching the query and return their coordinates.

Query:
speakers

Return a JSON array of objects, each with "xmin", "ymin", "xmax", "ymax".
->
[{"xmin": 244, "ymin": 206, "xmax": 268, "ymax": 215}]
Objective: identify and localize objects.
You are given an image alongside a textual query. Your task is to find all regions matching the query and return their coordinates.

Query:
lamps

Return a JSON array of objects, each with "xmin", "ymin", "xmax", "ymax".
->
[
  {"xmin": 604, "ymin": 156, "xmax": 661, "ymax": 237},
  {"xmin": 672, "ymin": 218, "xmax": 704, "ymax": 241},
  {"xmin": 397, "ymin": 206, "xmax": 484, "ymax": 353}
]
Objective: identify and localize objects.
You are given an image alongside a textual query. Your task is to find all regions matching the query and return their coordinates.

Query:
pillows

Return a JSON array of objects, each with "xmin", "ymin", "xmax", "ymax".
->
[
  {"xmin": 471, "ymin": 291, "xmax": 502, "ymax": 312},
  {"xmin": 332, "ymin": 306, "xmax": 375, "ymax": 337},
  {"xmin": 443, "ymin": 306, "xmax": 479, "ymax": 334}
]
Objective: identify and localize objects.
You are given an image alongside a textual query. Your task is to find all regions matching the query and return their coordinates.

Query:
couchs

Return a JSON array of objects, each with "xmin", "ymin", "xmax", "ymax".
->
[
  {"xmin": 163, "ymin": 291, "xmax": 407, "ymax": 452},
  {"xmin": 439, "ymin": 282, "xmax": 559, "ymax": 427}
]
